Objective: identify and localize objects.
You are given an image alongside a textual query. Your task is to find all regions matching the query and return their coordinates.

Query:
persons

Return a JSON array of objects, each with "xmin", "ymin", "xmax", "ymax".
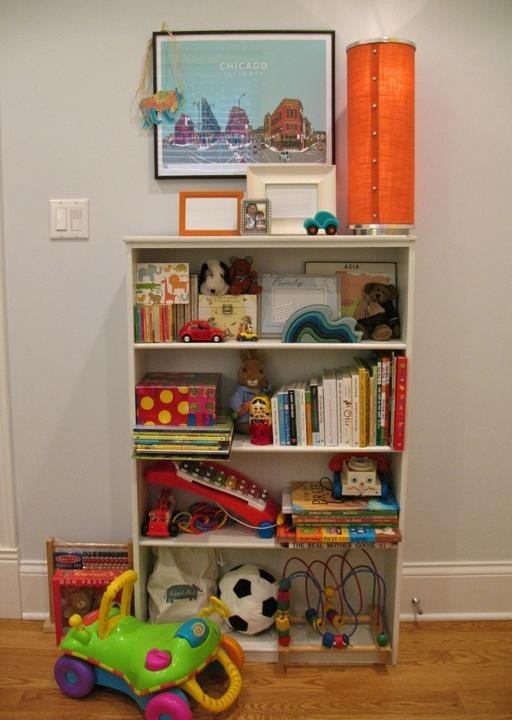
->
[
  {"xmin": 249, "ymin": 396, "xmax": 273, "ymax": 446},
  {"xmin": 244, "ymin": 203, "xmax": 266, "ymax": 231}
]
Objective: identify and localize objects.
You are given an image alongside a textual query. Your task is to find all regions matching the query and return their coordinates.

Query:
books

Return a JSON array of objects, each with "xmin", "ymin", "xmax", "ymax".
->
[
  {"xmin": 131, "ymin": 408, "xmax": 235, "ymax": 462},
  {"xmin": 133, "ymin": 263, "xmax": 198, "ymax": 344},
  {"xmin": 276, "ymin": 480, "xmax": 402, "ymax": 551},
  {"xmin": 271, "ymin": 349, "xmax": 408, "ymax": 451}
]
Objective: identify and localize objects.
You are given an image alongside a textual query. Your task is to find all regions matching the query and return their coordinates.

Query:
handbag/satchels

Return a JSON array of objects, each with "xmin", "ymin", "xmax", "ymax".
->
[{"xmin": 147, "ymin": 547, "xmax": 232, "ymax": 635}]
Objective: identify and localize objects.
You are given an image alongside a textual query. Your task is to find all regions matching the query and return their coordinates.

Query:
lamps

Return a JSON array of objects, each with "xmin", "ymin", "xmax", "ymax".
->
[{"xmin": 347, "ymin": 35, "xmax": 416, "ymax": 236}]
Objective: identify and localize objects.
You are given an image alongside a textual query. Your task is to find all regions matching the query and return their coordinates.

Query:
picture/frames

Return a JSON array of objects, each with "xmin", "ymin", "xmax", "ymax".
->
[
  {"xmin": 153, "ymin": 29, "xmax": 335, "ymax": 180},
  {"xmin": 240, "ymin": 198, "xmax": 272, "ymax": 236},
  {"xmin": 179, "ymin": 191, "xmax": 244, "ymax": 235}
]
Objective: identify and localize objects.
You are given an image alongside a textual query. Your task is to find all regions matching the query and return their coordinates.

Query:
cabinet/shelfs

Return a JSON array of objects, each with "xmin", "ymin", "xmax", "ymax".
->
[{"xmin": 124, "ymin": 235, "xmax": 418, "ymax": 667}]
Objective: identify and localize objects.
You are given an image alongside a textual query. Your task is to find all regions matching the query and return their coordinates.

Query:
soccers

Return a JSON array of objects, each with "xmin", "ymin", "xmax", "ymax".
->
[{"xmin": 216, "ymin": 563, "xmax": 280, "ymax": 634}]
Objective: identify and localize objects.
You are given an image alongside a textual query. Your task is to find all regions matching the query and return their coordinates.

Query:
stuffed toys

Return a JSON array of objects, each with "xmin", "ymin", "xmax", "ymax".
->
[
  {"xmin": 230, "ymin": 349, "xmax": 274, "ymax": 434},
  {"xmin": 59, "ymin": 586, "xmax": 101, "ymax": 628},
  {"xmin": 198, "ymin": 258, "xmax": 230, "ymax": 295},
  {"xmin": 226, "ymin": 256, "xmax": 263, "ymax": 295},
  {"xmin": 354, "ymin": 282, "xmax": 400, "ymax": 340}
]
